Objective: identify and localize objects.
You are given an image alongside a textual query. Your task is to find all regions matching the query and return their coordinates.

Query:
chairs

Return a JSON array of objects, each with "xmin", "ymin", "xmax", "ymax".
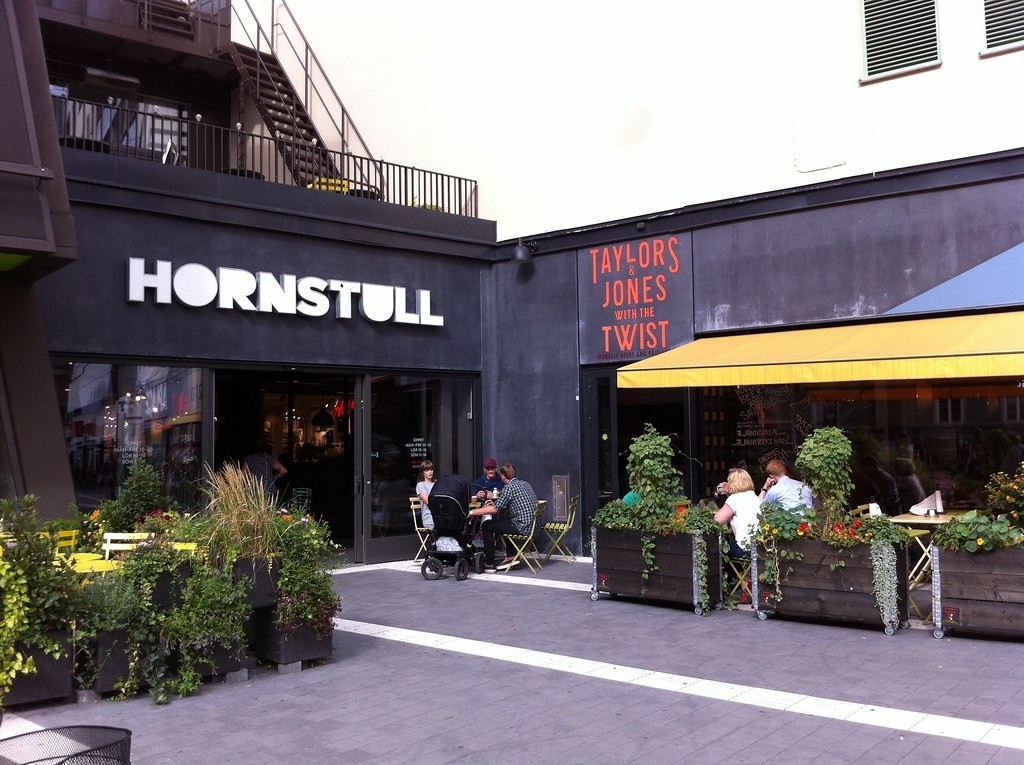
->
[
  {"xmin": 540, "ymin": 494, "xmax": 580, "ymax": 565},
  {"xmin": 849, "ymin": 503, "xmax": 869, "ymax": 520},
  {"xmin": 409, "ymin": 496, "xmax": 431, "ymax": 561},
  {"xmin": 167, "ymin": 543, "xmax": 198, "ymax": 560},
  {"xmin": 502, "ymin": 500, "xmax": 547, "ymax": 575},
  {"xmin": 35, "ymin": 529, "xmax": 80, "ymax": 558},
  {"xmin": 100, "ymin": 532, "xmax": 155, "ymax": 560}
]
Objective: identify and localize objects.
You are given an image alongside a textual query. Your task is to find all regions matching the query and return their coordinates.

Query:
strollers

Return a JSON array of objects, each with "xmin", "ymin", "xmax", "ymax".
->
[{"xmin": 421, "ymin": 474, "xmax": 483, "ymax": 581}]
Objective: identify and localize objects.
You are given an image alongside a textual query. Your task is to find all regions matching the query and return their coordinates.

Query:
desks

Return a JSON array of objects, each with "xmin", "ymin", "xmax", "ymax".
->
[
  {"xmin": 69, "ymin": 552, "xmax": 125, "ymax": 578},
  {"xmin": 887, "ymin": 509, "xmax": 976, "ymax": 592}
]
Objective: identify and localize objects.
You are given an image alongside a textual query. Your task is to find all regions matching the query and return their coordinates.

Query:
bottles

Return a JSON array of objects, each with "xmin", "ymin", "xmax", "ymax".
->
[
  {"xmin": 481, "ymin": 487, "xmax": 487, "ymax": 506},
  {"xmin": 492, "ymin": 488, "xmax": 498, "ymax": 498}
]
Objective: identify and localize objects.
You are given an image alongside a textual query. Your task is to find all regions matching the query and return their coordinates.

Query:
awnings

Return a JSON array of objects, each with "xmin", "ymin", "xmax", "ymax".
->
[{"xmin": 616, "ymin": 311, "xmax": 1024, "ymax": 388}]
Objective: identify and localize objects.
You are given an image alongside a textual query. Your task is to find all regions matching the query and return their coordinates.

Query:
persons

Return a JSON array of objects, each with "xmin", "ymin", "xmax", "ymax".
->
[
  {"xmin": 1007, "ymin": 432, "xmax": 1024, "ymax": 472},
  {"xmin": 859, "ymin": 455, "xmax": 902, "ymax": 517},
  {"xmin": 714, "ymin": 468, "xmax": 762, "ymax": 557},
  {"xmin": 470, "ymin": 463, "xmax": 542, "ymax": 572},
  {"xmin": 949, "ymin": 426, "xmax": 984, "ymax": 479},
  {"xmin": 372, "ymin": 461, "xmax": 410, "ymax": 522},
  {"xmin": 416, "ymin": 460, "xmax": 437, "ymax": 529},
  {"xmin": 615, "ymin": 482, "xmax": 641, "ymax": 505},
  {"xmin": 241, "ymin": 440, "xmax": 288, "ymax": 500},
  {"xmin": 893, "ymin": 457, "xmax": 925, "ymax": 514},
  {"xmin": 469, "ymin": 458, "xmax": 506, "ymax": 502},
  {"xmin": 758, "ymin": 460, "xmax": 812, "ymax": 516}
]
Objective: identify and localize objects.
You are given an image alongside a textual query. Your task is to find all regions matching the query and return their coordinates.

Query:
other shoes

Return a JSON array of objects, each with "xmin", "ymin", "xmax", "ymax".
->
[{"xmin": 739, "ymin": 582, "xmax": 753, "ymax": 604}]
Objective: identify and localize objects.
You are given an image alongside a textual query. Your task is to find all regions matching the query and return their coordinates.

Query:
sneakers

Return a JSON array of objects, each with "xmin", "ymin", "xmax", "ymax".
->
[
  {"xmin": 471, "ymin": 561, "xmax": 497, "ymax": 574},
  {"xmin": 496, "ymin": 556, "xmax": 520, "ymax": 570}
]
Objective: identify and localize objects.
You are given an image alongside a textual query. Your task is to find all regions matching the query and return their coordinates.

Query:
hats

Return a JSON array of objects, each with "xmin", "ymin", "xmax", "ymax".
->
[{"xmin": 483, "ymin": 458, "xmax": 497, "ymax": 468}]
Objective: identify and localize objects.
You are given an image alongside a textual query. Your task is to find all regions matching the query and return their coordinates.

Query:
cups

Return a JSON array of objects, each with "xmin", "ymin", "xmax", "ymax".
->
[{"xmin": 471, "ymin": 496, "xmax": 477, "ymax": 505}]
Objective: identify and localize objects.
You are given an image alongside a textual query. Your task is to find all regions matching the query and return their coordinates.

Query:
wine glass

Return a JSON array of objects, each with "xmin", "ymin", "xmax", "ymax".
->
[{"xmin": 712, "ymin": 482, "xmax": 728, "ymax": 498}]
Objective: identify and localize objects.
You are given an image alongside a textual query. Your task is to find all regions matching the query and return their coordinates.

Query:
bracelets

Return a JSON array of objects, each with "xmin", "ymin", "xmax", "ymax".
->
[{"xmin": 761, "ymin": 488, "xmax": 772, "ymax": 492}]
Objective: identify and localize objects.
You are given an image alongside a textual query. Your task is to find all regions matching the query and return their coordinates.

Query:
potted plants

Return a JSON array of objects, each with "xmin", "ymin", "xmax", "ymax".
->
[
  {"xmin": 931, "ymin": 461, "xmax": 1024, "ymax": 642},
  {"xmin": 0, "ymin": 493, "xmax": 74, "ymax": 706},
  {"xmin": 248, "ymin": 588, "xmax": 339, "ymax": 665},
  {"xmin": 748, "ymin": 425, "xmax": 911, "ymax": 636},
  {"xmin": 590, "ymin": 422, "xmax": 724, "ymax": 615},
  {"xmin": 196, "ymin": 459, "xmax": 280, "ymax": 606},
  {"xmin": 86, "ymin": 571, "xmax": 164, "ymax": 694}
]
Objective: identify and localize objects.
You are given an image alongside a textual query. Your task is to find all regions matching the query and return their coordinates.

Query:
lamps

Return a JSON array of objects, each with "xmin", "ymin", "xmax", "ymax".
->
[
  {"xmin": 511, "ymin": 238, "xmax": 540, "ymax": 264},
  {"xmin": 311, "ymin": 396, "xmax": 335, "ymax": 427}
]
